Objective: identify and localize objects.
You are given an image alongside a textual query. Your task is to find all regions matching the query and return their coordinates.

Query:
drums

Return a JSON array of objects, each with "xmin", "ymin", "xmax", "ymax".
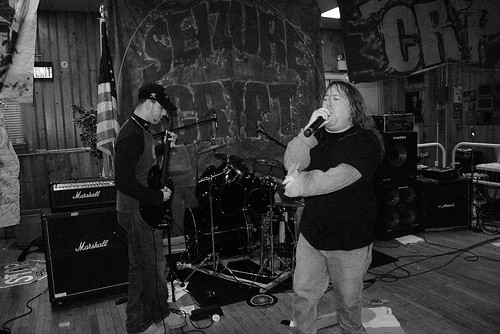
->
[{"xmin": 183, "ymin": 164, "xmax": 296, "ymax": 266}]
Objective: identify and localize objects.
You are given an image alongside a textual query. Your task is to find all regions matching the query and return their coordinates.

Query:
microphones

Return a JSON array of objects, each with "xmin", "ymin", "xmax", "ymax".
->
[
  {"xmin": 256, "ymin": 121, "xmax": 259, "ymax": 138},
  {"xmin": 213, "ymin": 110, "xmax": 218, "ymax": 130},
  {"xmin": 304, "ymin": 114, "xmax": 329, "ymax": 137}
]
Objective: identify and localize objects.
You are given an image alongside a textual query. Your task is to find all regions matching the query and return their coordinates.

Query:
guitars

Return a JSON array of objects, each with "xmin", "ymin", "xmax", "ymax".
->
[{"xmin": 138, "ymin": 129, "xmax": 174, "ymax": 230}]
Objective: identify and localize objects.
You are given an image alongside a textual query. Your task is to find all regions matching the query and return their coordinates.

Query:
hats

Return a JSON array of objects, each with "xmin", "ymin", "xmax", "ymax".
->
[{"xmin": 139, "ymin": 84, "xmax": 177, "ymax": 112}]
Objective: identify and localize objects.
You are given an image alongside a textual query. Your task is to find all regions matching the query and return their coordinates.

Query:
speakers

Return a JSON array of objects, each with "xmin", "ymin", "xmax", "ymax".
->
[
  {"xmin": 374, "ymin": 131, "xmax": 472, "ymax": 240},
  {"xmin": 40, "ymin": 204, "xmax": 131, "ymax": 306}
]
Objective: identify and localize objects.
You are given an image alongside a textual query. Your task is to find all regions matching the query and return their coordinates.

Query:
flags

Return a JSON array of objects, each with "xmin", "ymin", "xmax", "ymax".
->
[{"xmin": 95, "ymin": 18, "xmax": 120, "ymax": 176}]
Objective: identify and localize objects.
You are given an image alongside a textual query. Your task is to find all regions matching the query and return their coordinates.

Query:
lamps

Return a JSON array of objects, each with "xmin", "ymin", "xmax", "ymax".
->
[{"xmin": 318, "ymin": 0, "xmax": 341, "ymax": 19}]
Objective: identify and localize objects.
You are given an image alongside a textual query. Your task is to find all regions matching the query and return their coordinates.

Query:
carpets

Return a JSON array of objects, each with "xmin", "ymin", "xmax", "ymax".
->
[{"xmin": 177, "ymin": 240, "xmax": 399, "ymax": 307}]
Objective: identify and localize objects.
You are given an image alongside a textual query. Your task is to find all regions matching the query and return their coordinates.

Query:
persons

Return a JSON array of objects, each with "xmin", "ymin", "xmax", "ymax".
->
[
  {"xmin": 113, "ymin": 83, "xmax": 186, "ymax": 334},
  {"xmin": 282, "ymin": 80, "xmax": 390, "ymax": 334}
]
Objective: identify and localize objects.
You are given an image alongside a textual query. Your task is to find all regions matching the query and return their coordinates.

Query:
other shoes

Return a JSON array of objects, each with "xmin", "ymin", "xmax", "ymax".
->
[
  {"xmin": 157, "ymin": 311, "xmax": 185, "ymax": 329},
  {"xmin": 140, "ymin": 322, "xmax": 170, "ymax": 334}
]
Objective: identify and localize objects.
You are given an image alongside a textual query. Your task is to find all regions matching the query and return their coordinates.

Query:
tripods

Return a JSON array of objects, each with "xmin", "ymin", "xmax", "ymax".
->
[{"xmin": 180, "ymin": 168, "xmax": 294, "ymax": 289}]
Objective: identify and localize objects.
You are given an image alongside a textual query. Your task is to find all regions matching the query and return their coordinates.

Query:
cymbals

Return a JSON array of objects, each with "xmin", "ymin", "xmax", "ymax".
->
[
  {"xmin": 197, "ymin": 143, "xmax": 233, "ymax": 155},
  {"xmin": 211, "ymin": 151, "xmax": 243, "ymax": 164},
  {"xmin": 252, "ymin": 158, "xmax": 289, "ymax": 179},
  {"xmin": 273, "ymin": 201, "xmax": 306, "ymax": 211}
]
